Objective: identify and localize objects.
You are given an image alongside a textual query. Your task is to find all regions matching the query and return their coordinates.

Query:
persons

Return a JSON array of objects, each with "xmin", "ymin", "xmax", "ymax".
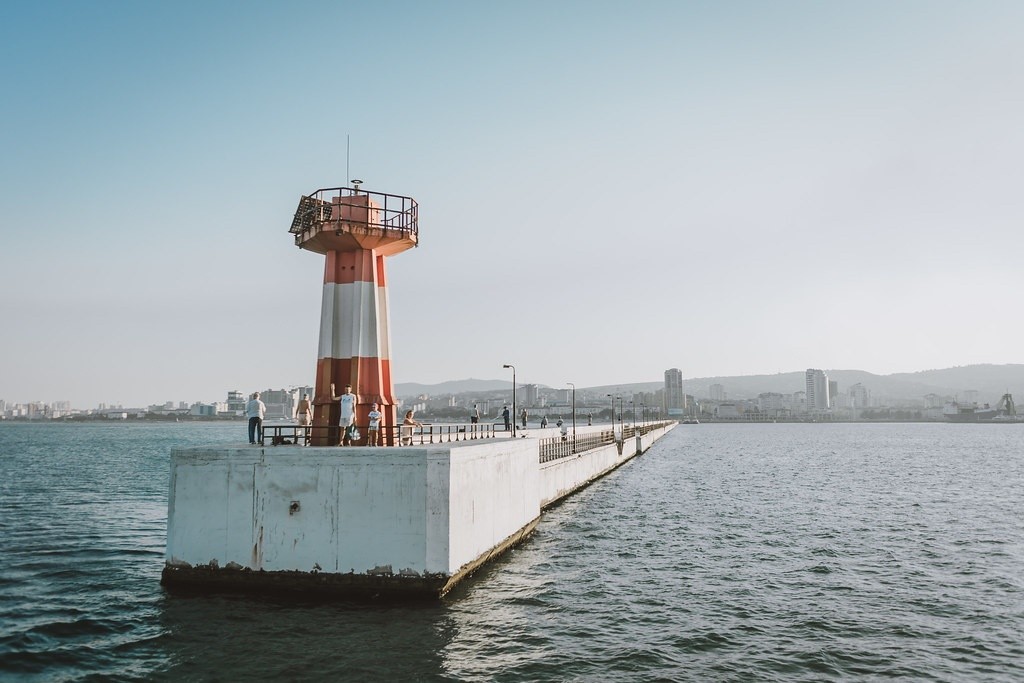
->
[
  {"xmin": 588, "ymin": 412, "xmax": 593, "ymax": 425},
  {"xmin": 470, "ymin": 405, "xmax": 479, "ymax": 432},
  {"xmin": 557, "ymin": 415, "xmax": 563, "ymax": 427},
  {"xmin": 368, "ymin": 403, "xmax": 381, "ymax": 447},
  {"xmin": 618, "ymin": 414, "xmax": 621, "ymax": 421},
  {"xmin": 331, "ymin": 383, "xmax": 357, "ymax": 446},
  {"xmin": 521, "ymin": 408, "xmax": 527, "ymax": 426},
  {"xmin": 295, "ymin": 393, "xmax": 312, "ymax": 436},
  {"xmin": 502, "ymin": 406, "xmax": 510, "ymax": 431},
  {"xmin": 402, "ymin": 410, "xmax": 423, "ymax": 445},
  {"xmin": 246, "ymin": 392, "xmax": 266, "ymax": 444},
  {"xmin": 541, "ymin": 416, "xmax": 547, "ymax": 428}
]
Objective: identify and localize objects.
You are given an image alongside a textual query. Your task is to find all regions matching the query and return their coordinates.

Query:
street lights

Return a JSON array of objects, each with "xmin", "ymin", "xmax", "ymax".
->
[
  {"xmin": 502, "ymin": 364, "xmax": 517, "ymax": 437},
  {"xmin": 644, "ymin": 406, "xmax": 648, "ymax": 425},
  {"xmin": 607, "ymin": 394, "xmax": 614, "ymax": 436},
  {"xmin": 616, "ymin": 396, "xmax": 624, "ymax": 432},
  {"xmin": 567, "ymin": 383, "xmax": 577, "ymax": 452},
  {"xmin": 650, "ymin": 407, "xmax": 653, "ymax": 425},
  {"xmin": 640, "ymin": 403, "xmax": 645, "ymax": 427},
  {"xmin": 629, "ymin": 402, "xmax": 635, "ymax": 427}
]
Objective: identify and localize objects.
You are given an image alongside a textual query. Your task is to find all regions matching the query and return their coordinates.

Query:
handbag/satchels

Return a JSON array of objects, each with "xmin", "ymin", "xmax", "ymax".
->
[{"xmin": 347, "ymin": 423, "xmax": 361, "ymax": 440}]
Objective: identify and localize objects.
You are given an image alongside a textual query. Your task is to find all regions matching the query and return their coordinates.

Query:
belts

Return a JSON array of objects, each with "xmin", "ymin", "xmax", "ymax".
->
[{"xmin": 300, "ymin": 412, "xmax": 307, "ymax": 414}]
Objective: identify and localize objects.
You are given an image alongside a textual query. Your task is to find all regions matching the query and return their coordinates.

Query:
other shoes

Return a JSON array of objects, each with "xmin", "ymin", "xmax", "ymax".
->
[
  {"xmin": 258, "ymin": 442, "xmax": 263, "ymax": 444},
  {"xmin": 250, "ymin": 442, "xmax": 256, "ymax": 444},
  {"xmin": 338, "ymin": 443, "xmax": 343, "ymax": 446},
  {"xmin": 348, "ymin": 441, "xmax": 351, "ymax": 446}
]
